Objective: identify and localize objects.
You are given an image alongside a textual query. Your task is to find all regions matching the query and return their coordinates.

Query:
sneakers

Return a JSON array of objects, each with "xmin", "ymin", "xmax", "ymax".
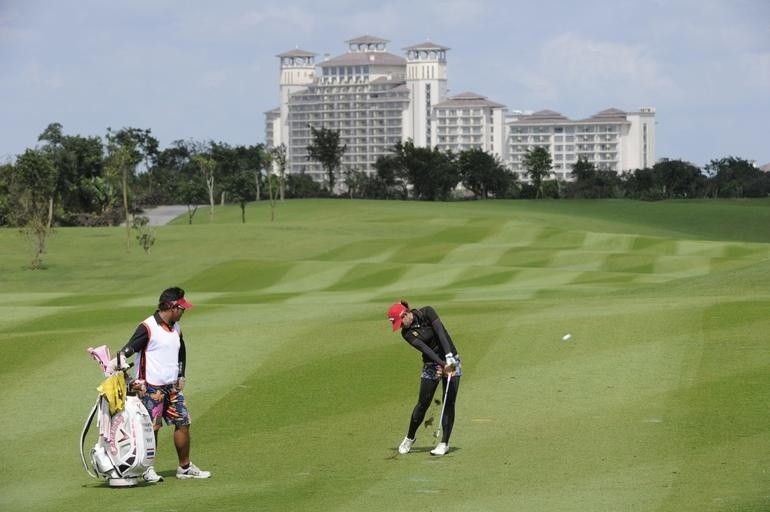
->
[
  {"xmin": 399, "ymin": 435, "xmax": 416, "ymax": 454},
  {"xmin": 176, "ymin": 461, "xmax": 211, "ymax": 479},
  {"xmin": 430, "ymin": 443, "xmax": 449, "ymax": 455},
  {"xmin": 143, "ymin": 467, "xmax": 164, "ymax": 483}
]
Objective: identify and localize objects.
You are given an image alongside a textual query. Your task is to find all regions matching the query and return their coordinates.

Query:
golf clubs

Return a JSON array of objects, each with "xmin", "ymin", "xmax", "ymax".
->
[{"xmin": 432, "ymin": 371, "xmax": 453, "ymax": 438}]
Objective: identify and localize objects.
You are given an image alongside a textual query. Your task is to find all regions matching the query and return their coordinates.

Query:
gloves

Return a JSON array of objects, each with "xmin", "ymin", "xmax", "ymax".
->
[{"xmin": 443, "ymin": 353, "xmax": 456, "ymax": 376}]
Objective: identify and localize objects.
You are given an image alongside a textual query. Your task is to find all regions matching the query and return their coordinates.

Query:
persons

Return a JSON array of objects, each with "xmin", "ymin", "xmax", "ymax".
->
[
  {"xmin": 386, "ymin": 300, "xmax": 463, "ymax": 456},
  {"xmin": 116, "ymin": 287, "xmax": 212, "ymax": 483}
]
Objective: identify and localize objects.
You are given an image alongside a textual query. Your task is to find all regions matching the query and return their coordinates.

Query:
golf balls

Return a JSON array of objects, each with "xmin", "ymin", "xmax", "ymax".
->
[{"xmin": 563, "ymin": 334, "xmax": 570, "ymax": 341}]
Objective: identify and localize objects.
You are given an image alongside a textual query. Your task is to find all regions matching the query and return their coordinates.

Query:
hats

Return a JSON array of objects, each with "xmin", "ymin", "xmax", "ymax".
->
[
  {"xmin": 388, "ymin": 304, "xmax": 406, "ymax": 331},
  {"xmin": 159, "ymin": 297, "xmax": 193, "ymax": 310}
]
